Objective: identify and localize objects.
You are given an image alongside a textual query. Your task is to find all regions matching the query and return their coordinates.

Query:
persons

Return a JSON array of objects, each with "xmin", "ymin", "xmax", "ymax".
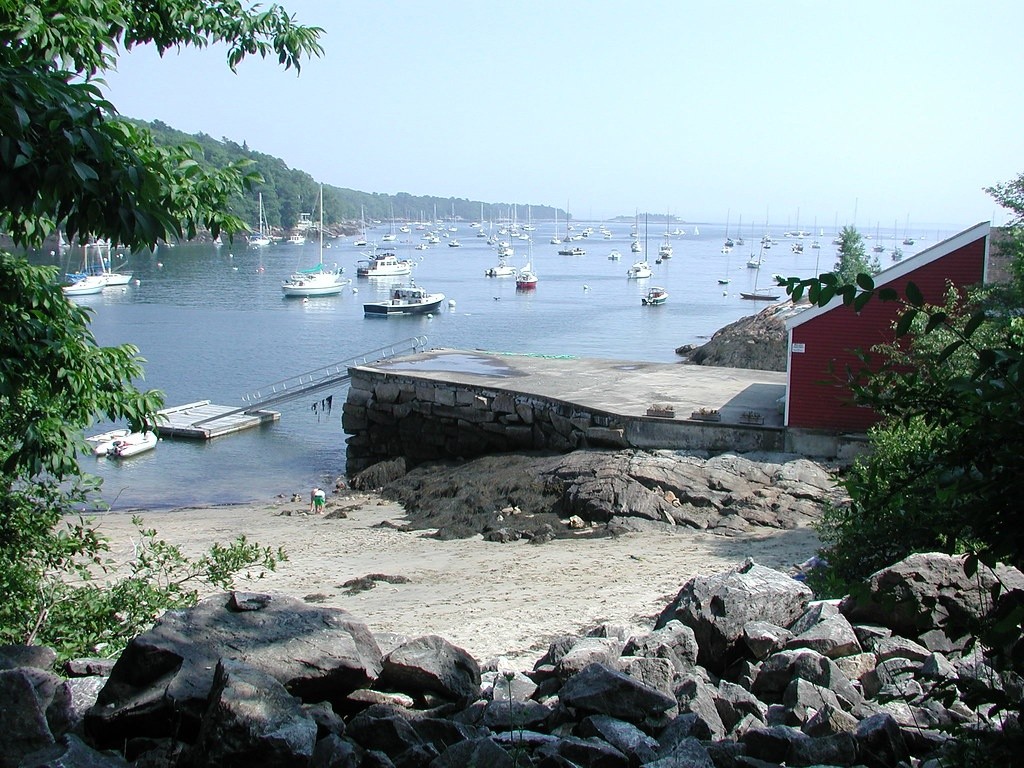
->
[{"xmin": 394, "ymin": 290, "xmax": 400, "ymax": 299}]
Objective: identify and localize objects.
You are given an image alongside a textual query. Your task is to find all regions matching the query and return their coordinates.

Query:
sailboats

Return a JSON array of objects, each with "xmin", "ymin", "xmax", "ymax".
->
[
  {"xmin": 353, "ymin": 201, "xmax": 915, "ymax": 279},
  {"xmin": 514, "ymin": 228, "xmax": 538, "ymax": 289},
  {"xmin": 280, "ymin": 182, "xmax": 348, "ymax": 295},
  {"xmin": 244, "ymin": 192, "xmax": 305, "ymax": 246},
  {"xmin": 740, "ymin": 241, "xmax": 781, "ymax": 301},
  {"xmin": 59, "ymin": 230, "xmax": 133, "ymax": 296}
]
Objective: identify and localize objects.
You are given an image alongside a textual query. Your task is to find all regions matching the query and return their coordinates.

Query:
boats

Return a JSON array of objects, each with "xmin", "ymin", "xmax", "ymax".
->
[
  {"xmin": 84, "ymin": 429, "xmax": 157, "ymax": 458},
  {"xmin": 717, "ymin": 279, "xmax": 731, "ymax": 285},
  {"xmin": 642, "ymin": 287, "xmax": 669, "ymax": 306},
  {"xmin": 363, "ymin": 277, "xmax": 445, "ymax": 318},
  {"xmin": 213, "ymin": 235, "xmax": 224, "ymax": 246}
]
